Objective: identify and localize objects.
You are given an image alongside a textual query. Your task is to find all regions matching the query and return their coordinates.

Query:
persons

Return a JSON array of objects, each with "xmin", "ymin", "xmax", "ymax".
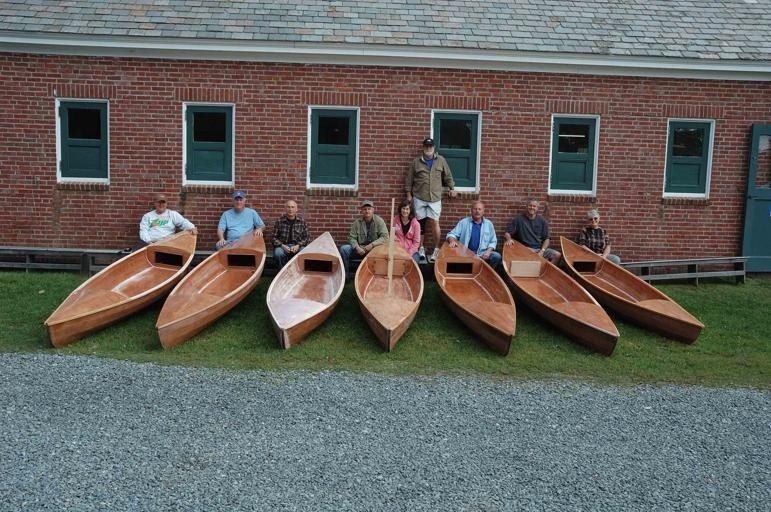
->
[
  {"xmin": 503, "ymin": 200, "xmax": 561, "ymax": 266},
  {"xmin": 340, "ymin": 200, "xmax": 390, "ymax": 284},
  {"xmin": 216, "ymin": 192, "xmax": 266, "ymax": 251},
  {"xmin": 404, "ymin": 138, "xmax": 456, "ymax": 262},
  {"xmin": 270, "ymin": 200, "xmax": 307, "ymax": 271},
  {"xmin": 392, "ymin": 199, "xmax": 421, "ymax": 266},
  {"xmin": 446, "ymin": 202, "xmax": 501, "ymax": 270},
  {"xmin": 132, "ymin": 194, "xmax": 198, "ymax": 254},
  {"xmin": 579, "ymin": 210, "xmax": 621, "ymax": 265}
]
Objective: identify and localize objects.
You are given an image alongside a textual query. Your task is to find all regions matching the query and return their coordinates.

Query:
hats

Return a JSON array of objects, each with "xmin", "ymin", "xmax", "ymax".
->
[
  {"xmin": 361, "ymin": 200, "xmax": 373, "ymax": 207},
  {"xmin": 232, "ymin": 191, "xmax": 245, "ymax": 199},
  {"xmin": 423, "ymin": 138, "xmax": 435, "ymax": 145},
  {"xmin": 154, "ymin": 194, "xmax": 166, "ymax": 202}
]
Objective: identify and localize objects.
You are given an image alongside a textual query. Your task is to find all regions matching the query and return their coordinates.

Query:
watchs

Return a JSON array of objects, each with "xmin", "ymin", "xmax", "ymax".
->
[{"xmin": 540, "ymin": 247, "xmax": 546, "ymax": 252}]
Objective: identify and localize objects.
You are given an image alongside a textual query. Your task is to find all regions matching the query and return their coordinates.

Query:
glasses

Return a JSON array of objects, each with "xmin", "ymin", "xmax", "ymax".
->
[{"xmin": 590, "ymin": 217, "xmax": 598, "ymax": 220}]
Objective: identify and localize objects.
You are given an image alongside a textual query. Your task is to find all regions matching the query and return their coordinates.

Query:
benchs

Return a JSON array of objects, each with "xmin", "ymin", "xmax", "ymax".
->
[
  {"xmin": 86, "ymin": 249, "xmax": 216, "ymax": 276},
  {"xmin": 621, "ymin": 256, "xmax": 752, "ymax": 287},
  {"xmin": 0, "ymin": 246, "xmax": 86, "ymax": 274},
  {"xmin": 262, "ymin": 250, "xmax": 428, "ymax": 278}
]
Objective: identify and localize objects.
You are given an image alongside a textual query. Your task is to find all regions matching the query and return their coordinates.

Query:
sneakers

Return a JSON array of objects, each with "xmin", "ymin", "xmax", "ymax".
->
[
  {"xmin": 418, "ymin": 246, "xmax": 425, "ymax": 258},
  {"xmin": 430, "ymin": 248, "xmax": 439, "ymax": 261}
]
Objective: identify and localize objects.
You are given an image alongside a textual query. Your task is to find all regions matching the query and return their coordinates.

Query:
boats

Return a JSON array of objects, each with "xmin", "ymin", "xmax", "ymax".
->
[
  {"xmin": 354, "ymin": 243, "xmax": 425, "ymax": 351},
  {"xmin": 154, "ymin": 232, "xmax": 267, "ymax": 351},
  {"xmin": 44, "ymin": 230, "xmax": 197, "ymax": 348},
  {"xmin": 502, "ymin": 239, "xmax": 620, "ymax": 356},
  {"xmin": 434, "ymin": 238, "xmax": 519, "ymax": 357},
  {"xmin": 265, "ymin": 231, "xmax": 346, "ymax": 350},
  {"xmin": 559, "ymin": 235, "xmax": 707, "ymax": 346}
]
